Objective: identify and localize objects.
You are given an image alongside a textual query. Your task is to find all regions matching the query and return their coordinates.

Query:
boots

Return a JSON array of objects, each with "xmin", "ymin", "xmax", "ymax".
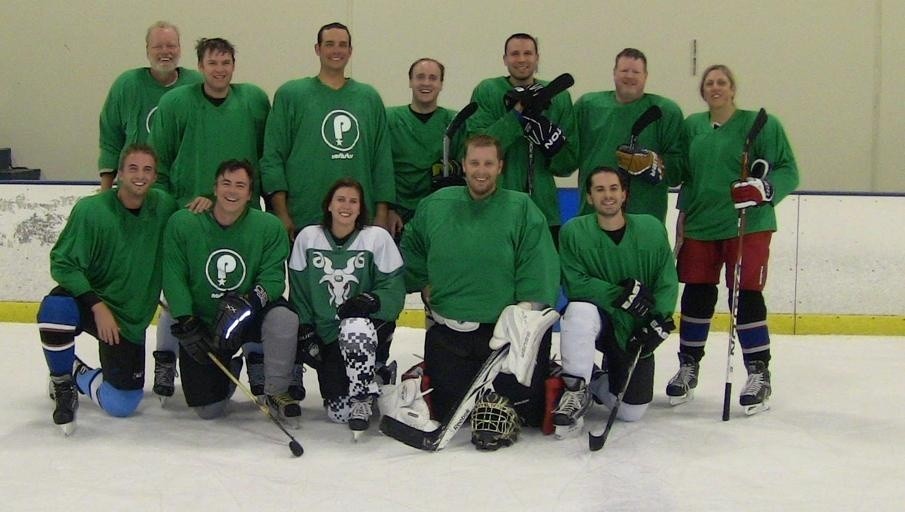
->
[
  {"xmin": 152, "ymin": 351, "xmax": 176, "ymax": 397},
  {"xmin": 739, "ymin": 360, "xmax": 772, "ymax": 406},
  {"xmin": 553, "ymin": 362, "xmax": 609, "ymax": 425},
  {"xmin": 49, "ymin": 355, "xmax": 87, "ymax": 425},
  {"xmin": 666, "ymin": 352, "xmax": 699, "ymax": 394}
]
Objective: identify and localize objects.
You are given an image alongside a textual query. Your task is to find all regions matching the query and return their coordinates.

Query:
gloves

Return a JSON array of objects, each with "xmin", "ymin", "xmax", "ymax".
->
[
  {"xmin": 629, "ymin": 313, "xmax": 676, "ymax": 359},
  {"xmin": 616, "ymin": 277, "xmax": 656, "ymax": 318},
  {"xmin": 431, "ymin": 159, "xmax": 466, "ymax": 190},
  {"xmin": 731, "ymin": 177, "xmax": 775, "ymax": 210},
  {"xmin": 338, "ymin": 291, "xmax": 380, "ymax": 318},
  {"xmin": 520, "ymin": 115, "xmax": 566, "ymax": 158},
  {"xmin": 213, "ymin": 296, "xmax": 258, "ymax": 352},
  {"xmin": 172, "ymin": 320, "xmax": 219, "ymax": 366},
  {"xmin": 298, "ymin": 324, "xmax": 326, "ymax": 370},
  {"xmin": 614, "ymin": 144, "xmax": 665, "ymax": 185},
  {"xmin": 503, "ymin": 83, "xmax": 551, "ymax": 114}
]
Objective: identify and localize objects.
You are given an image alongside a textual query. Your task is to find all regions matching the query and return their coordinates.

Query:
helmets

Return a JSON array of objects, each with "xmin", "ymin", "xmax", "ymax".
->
[{"xmin": 471, "ymin": 394, "xmax": 520, "ymax": 450}]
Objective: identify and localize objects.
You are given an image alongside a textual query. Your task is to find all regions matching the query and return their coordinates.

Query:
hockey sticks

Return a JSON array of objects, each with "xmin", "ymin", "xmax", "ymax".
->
[
  {"xmin": 526, "ymin": 73, "xmax": 573, "ymax": 196},
  {"xmin": 588, "ymin": 344, "xmax": 643, "ymax": 451},
  {"xmin": 158, "ymin": 299, "xmax": 304, "ymax": 457},
  {"xmin": 444, "ymin": 101, "xmax": 477, "ymax": 180},
  {"xmin": 380, "ymin": 344, "xmax": 509, "ymax": 452},
  {"xmin": 722, "ymin": 109, "xmax": 767, "ymax": 421},
  {"xmin": 619, "ymin": 106, "xmax": 662, "ymax": 215}
]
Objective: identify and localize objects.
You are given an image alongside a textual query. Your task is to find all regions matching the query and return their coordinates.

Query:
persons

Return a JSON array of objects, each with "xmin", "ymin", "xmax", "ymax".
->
[
  {"xmin": 261, "ymin": 22, "xmax": 395, "ymax": 398},
  {"xmin": 100, "ymin": 22, "xmax": 202, "ymax": 200},
  {"xmin": 161, "ymin": 159, "xmax": 300, "ymax": 424},
  {"xmin": 573, "ymin": 47, "xmax": 685, "ymax": 225},
  {"xmin": 400, "ymin": 136, "xmax": 561, "ymax": 447},
  {"xmin": 289, "ymin": 178, "xmax": 405, "ymax": 432},
  {"xmin": 666, "ymin": 64, "xmax": 799, "ymax": 405},
  {"xmin": 469, "ymin": 31, "xmax": 580, "ymax": 249},
  {"xmin": 148, "ymin": 36, "xmax": 270, "ymax": 396},
  {"xmin": 36, "ymin": 145, "xmax": 210, "ymax": 424},
  {"xmin": 550, "ymin": 165, "xmax": 679, "ymax": 426},
  {"xmin": 375, "ymin": 57, "xmax": 466, "ymax": 377}
]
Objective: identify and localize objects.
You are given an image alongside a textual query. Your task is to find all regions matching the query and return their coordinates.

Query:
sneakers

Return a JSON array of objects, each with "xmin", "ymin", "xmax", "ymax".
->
[
  {"xmin": 349, "ymin": 396, "xmax": 372, "ymax": 430},
  {"xmin": 245, "ymin": 352, "xmax": 305, "ymax": 418}
]
